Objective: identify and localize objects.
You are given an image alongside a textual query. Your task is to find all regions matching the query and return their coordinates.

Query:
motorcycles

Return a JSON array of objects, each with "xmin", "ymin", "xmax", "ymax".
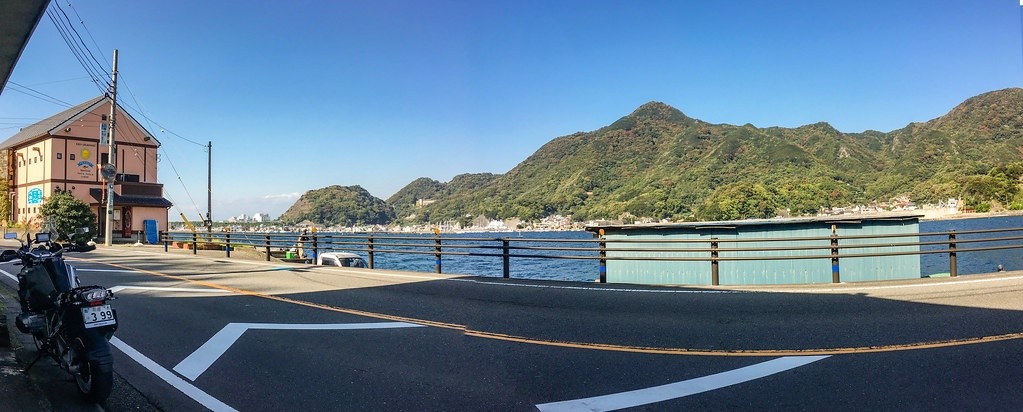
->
[{"xmin": 4, "ymin": 233, "xmax": 118, "ymax": 403}]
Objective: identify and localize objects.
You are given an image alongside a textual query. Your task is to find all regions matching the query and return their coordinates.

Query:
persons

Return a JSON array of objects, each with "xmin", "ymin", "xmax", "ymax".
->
[{"xmin": 297, "ymin": 228, "xmax": 311, "ymax": 258}]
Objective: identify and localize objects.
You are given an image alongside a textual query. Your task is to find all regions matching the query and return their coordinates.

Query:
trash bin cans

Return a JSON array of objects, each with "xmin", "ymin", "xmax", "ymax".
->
[{"xmin": 138, "ymin": 230, "xmax": 145, "ymax": 244}]
